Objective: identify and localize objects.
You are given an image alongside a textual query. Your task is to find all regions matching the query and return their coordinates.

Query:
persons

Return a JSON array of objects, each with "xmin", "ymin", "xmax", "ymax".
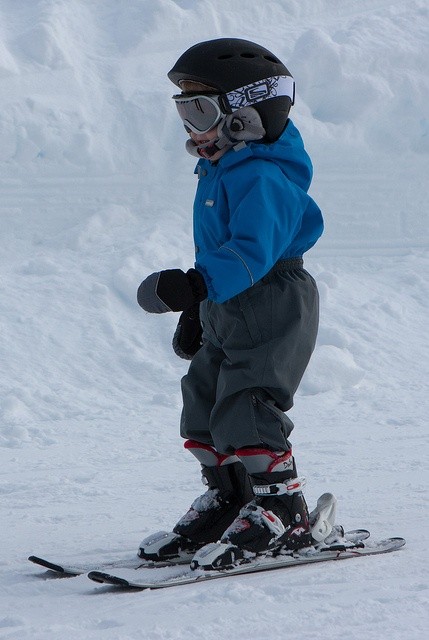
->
[{"xmin": 134, "ymin": 35, "xmax": 327, "ymax": 569}]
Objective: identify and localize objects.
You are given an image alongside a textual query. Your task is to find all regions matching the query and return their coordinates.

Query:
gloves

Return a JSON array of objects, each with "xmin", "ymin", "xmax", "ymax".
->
[
  {"xmin": 137, "ymin": 269, "xmax": 207, "ymax": 315},
  {"xmin": 171, "ymin": 300, "xmax": 203, "ymax": 359}
]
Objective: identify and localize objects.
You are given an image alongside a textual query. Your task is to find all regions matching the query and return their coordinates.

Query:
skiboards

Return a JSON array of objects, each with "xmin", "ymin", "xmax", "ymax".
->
[{"xmin": 26, "ymin": 529, "xmax": 406, "ymax": 586}]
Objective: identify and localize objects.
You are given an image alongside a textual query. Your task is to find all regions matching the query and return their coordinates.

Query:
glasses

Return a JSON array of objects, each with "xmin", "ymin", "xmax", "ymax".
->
[{"xmin": 171, "ymin": 76, "xmax": 294, "ymax": 135}]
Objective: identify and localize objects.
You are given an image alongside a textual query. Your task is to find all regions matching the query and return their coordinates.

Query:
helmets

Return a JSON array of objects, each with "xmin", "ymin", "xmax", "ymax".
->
[{"xmin": 167, "ymin": 37, "xmax": 294, "ymax": 144}]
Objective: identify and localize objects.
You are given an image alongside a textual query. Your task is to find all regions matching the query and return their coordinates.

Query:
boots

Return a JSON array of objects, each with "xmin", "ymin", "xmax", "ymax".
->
[
  {"xmin": 174, "ymin": 441, "xmax": 256, "ymax": 553},
  {"xmin": 217, "ymin": 447, "xmax": 313, "ymax": 559}
]
